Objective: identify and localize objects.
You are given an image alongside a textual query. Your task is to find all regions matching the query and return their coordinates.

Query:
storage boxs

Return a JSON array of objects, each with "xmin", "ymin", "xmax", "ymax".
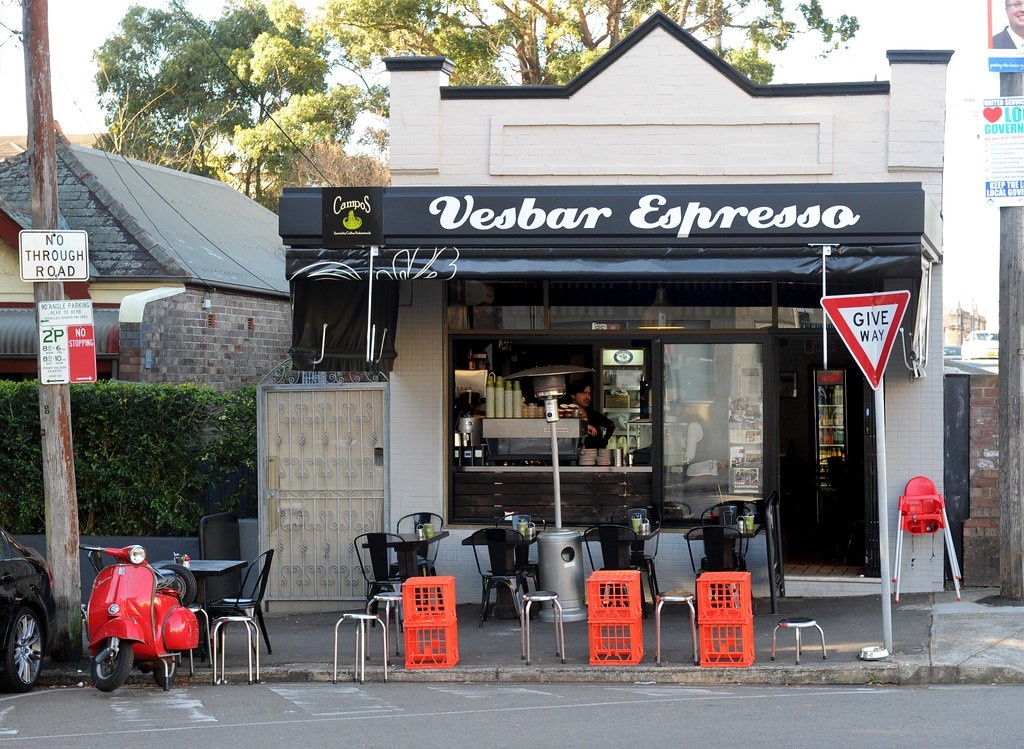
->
[
  {"xmin": 606, "ymin": 395, "xmax": 631, "ymax": 408},
  {"xmin": 696, "ymin": 571, "xmax": 758, "ymax": 668},
  {"xmin": 400, "ymin": 576, "xmax": 460, "ymax": 670},
  {"xmin": 585, "ymin": 570, "xmax": 645, "ymax": 666}
]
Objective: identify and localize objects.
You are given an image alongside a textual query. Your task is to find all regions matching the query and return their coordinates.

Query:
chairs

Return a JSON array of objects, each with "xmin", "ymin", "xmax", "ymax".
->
[
  {"xmin": 199, "ymin": 548, "xmax": 274, "ymax": 663},
  {"xmin": 814, "ymin": 457, "xmax": 833, "ymax": 524},
  {"xmin": 585, "ymin": 505, "xmax": 663, "ymax": 619},
  {"xmin": 353, "ymin": 511, "xmax": 444, "ymax": 633},
  {"xmin": 685, "ymin": 501, "xmax": 764, "ymax": 622},
  {"xmin": 892, "ymin": 476, "xmax": 962, "ymax": 602},
  {"xmin": 87, "ymin": 549, "xmax": 103, "ymax": 575},
  {"xmin": 471, "ymin": 512, "xmax": 546, "ymax": 628}
]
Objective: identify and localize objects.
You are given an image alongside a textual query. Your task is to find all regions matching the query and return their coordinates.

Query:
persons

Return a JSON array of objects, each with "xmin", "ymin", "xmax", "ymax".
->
[
  {"xmin": 684, "ymin": 413, "xmax": 709, "ymax": 466},
  {"xmin": 992, "ymin": 0, "xmax": 1024, "ymax": 49},
  {"xmin": 569, "ymin": 379, "xmax": 615, "ymax": 449}
]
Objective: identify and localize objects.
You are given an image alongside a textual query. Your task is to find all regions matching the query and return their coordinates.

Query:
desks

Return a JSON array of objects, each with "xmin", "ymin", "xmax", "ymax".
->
[
  {"xmin": 575, "ymin": 526, "xmax": 660, "ymax": 616},
  {"xmin": 146, "ymin": 558, "xmax": 248, "ymax": 655},
  {"xmin": 362, "ymin": 531, "xmax": 451, "ymax": 605},
  {"xmin": 681, "ymin": 524, "xmax": 762, "ymax": 577},
  {"xmin": 462, "ymin": 527, "xmax": 542, "ymax": 621}
]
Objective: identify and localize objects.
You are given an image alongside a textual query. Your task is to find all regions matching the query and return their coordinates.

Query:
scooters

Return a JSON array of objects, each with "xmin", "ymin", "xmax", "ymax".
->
[{"xmin": 79, "ymin": 544, "xmax": 199, "ymax": 692}]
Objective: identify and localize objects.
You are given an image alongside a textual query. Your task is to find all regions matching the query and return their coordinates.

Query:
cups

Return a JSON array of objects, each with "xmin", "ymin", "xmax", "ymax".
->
[
  {"xmin": 516, "ymin": 522, "xmax": 528, "ymax": 536},
  {"xmin": 744, "ymin": 515, "xmax": 754, "ymax": 532},
  {"xmin": 614, "ymin": 449, "xmax": 623, "ymax": 466},
  {"xmin": 485, "ymin": 374, "xmax": 523, "ymax": 419},
  {"xmin": 632, "ymin": 518, "xmax": 642, "ymax": 533},
  {"xmin": 424, "ymin": 524, "xmax": 434, "ymax": 538}
]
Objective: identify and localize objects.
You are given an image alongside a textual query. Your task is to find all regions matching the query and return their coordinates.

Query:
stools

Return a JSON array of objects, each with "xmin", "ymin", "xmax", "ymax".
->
[
  {"xmin": 365, "ymin": 592, "xmax": 418, "ymax": 661},
  {"xmin": 770, "ymin": 618, "xmax": 827, "ymax": 666},
  {"xmin": 178, "ymin": 603, "xmax": 212, "ymax": 678},
  {"xmin": 521, "ymin": 590, "xmax": 566, "ymax": 666},
  {"xmin": 655, "ymin": 590, "xmax": 699, "ymax": 666},
  {"xmin": 212, "ymin": 616, "xmax": 260, "ymax": 686},
  {"xmin": 333, "ymin": 614, "xmax": 389, "ymax": 686}
]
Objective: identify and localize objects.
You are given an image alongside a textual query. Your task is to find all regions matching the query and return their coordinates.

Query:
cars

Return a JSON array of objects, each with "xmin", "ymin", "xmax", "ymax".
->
[
  {"xmin": 0, "ymin": 527, "xmax": 56, "ymax": 693},
  {"xmin": 944, "ymin": 330, "xmax": 999, "ymax": 360}
]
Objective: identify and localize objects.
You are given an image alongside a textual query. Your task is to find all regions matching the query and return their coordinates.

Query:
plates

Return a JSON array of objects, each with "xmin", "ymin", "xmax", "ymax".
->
[{"xmin": 579, "ymin": 448, "xmax": 614, "ymax": 466}]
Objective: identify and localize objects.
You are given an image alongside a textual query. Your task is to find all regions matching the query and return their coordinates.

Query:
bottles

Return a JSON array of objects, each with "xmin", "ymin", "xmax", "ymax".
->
[
  {"xmin": 528, "ymin": 403, "xmax": 538, "ymax": 417},
  {"xmin": 735, "ymin": 515, "xmax": 747, "ymax": 534},
  {"xmin": 417, "ymin": 529, "xmax": 424, "ymax": 538},
  {"xmin": 528, "ymin": 528, "xmax": 533, "ymax": 536},
  {"xmin": 172, "ymin": 552, "xmax": 191, "ymax": 569}
]
